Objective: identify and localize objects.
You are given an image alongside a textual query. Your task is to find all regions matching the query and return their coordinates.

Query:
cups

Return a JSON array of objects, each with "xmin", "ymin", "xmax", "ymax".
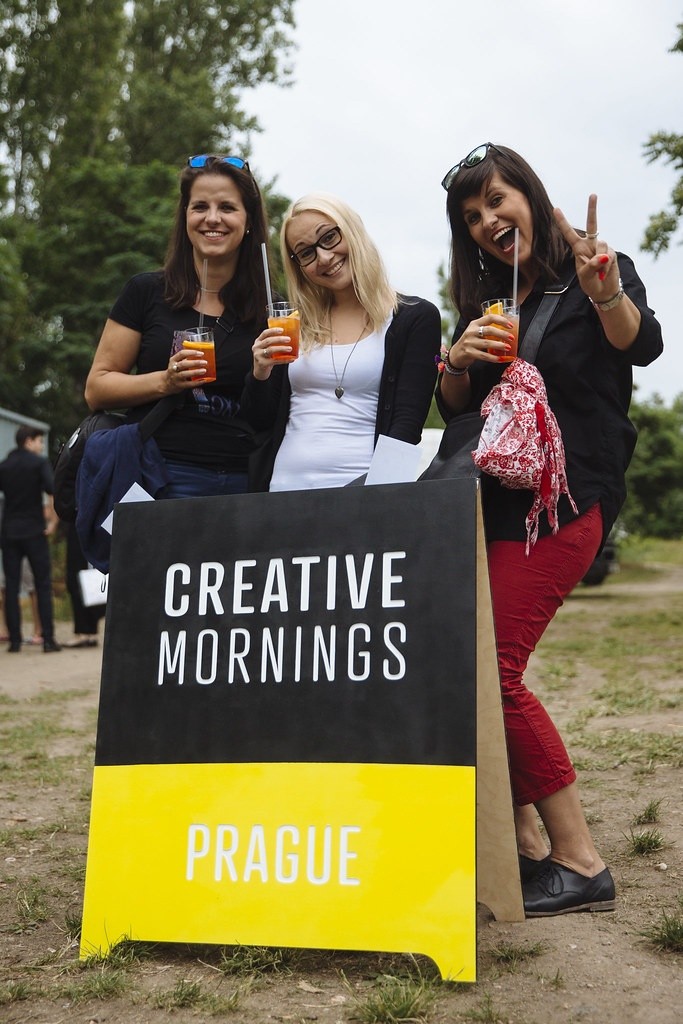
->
[
  {"xmin": 182, "ymin": 327, "xmax": 217, "ymax": 384},
  {"xmin": 266, "ymin": 301, "xmax": 302, "ymax": 361},
  {"xmin": 481, "ymin": 298, "xmax": 521, "ymax": 363}
]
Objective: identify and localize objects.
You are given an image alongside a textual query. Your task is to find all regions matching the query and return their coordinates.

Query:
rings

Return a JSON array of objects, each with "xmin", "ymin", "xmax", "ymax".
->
[
  {"xmin": 264, "ymin": 349, "xmax": 270, "ymax": 357},
  {"xmin": 479, "ymin": 327, "xmax": 484, "ymax": 337},
  {"xmin": 173, "ymin": 362, "xmax": 180, "ymax": 372},
  {"xmin": 586, "ymin": 232, "xmax": 599, "ymax": 238}
]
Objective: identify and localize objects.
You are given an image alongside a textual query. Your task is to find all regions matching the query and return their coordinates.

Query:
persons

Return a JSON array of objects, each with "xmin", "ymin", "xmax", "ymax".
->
[
  {"xmin": 435, "ymin": 142, "xmax": 665, "ymax": 917},
  {"xmin": 85, "ymin": 154, "xmax": 284, "ymax": 497},
  {"xmin": 0, "ymin": 426, "xmax": 107, "ymax": 653},
  {"xmin": 241, "ymin": 193, "xmax": 441, "ymax": 492}
]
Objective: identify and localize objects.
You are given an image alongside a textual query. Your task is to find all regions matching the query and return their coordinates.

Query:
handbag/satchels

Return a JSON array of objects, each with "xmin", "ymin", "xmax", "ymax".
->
[
  {"xmin": 417, "ymin": 412, "xmax": 487, "ymax": 481},
  {"xmin": 52, "ymin": 410, "xmax": 143, "ymax": 523}
]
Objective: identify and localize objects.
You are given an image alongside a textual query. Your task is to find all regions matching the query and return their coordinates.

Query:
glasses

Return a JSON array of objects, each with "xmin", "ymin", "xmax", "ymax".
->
[
  {"xmin": 289, "ymin": 226, "xmax": 345, "ymax": 268},
  {"xmin": 186, "ymin": 154, "xmax": 251, "ymax": 178},
  {"xmin": 440, "ymin": 140, "xmax": 511, "ymax": 193}
]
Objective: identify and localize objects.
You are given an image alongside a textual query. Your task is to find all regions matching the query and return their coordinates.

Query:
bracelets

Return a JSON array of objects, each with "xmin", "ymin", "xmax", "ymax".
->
[
  {"xmin": 589, "ymin": 278, "xmax": 623, "ymax": 311},
  {"xmin": 434, "ymin": 345, "xmax": 469, "ymax": 376}
]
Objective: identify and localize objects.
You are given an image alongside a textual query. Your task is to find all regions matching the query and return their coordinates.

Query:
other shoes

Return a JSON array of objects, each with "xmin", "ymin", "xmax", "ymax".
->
[
  {"xmin": 43, "ymin": 640, "xmax": 61, "ymax": 652},
  {"xmin": 7, "ymin": 641, "xmax": 19, "ymax": 652},
  {"xmin": 65, "ymin": 635, "xmax": 97, "ymax": 648}
]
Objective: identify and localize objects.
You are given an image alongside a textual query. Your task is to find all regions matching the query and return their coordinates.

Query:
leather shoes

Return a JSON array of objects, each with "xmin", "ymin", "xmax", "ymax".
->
[{"xmin": 519, "ymin": 851, "xmax": 616, "ymax": 916}]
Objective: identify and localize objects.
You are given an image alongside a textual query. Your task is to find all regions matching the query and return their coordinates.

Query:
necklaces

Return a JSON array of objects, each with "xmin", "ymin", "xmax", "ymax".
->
[
  {"xmin": 197, "ymin": 286, "xmax": 219, "ymax": 293},
  {"xmin": 329, "ymin": 307, "xmax": 370, "ymax": 399}
]
